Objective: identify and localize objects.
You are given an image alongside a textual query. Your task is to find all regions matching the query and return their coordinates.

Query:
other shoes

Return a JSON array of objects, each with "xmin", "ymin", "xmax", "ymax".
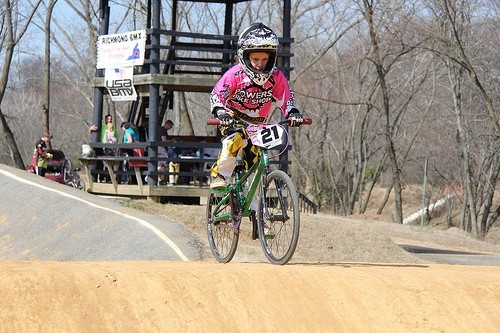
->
[
  {"xmin": 207, "ymin": 176, "xmax": 227, "ymax": 189},
  {"xmin": 263, "ymin": 223, "xmax": 275, "ymax": 235}
]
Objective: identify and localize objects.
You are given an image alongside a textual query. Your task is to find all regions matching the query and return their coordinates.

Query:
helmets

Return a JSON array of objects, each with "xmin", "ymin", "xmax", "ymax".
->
[{"xmin": 236, "ymin": 23, "xmax": 279, "ymax": 86}]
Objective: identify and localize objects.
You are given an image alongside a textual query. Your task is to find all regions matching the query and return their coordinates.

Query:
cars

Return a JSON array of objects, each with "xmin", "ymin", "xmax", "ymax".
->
[{"xmin": 43, "ymin": 149, "xmax": 67, "ymax": 184}]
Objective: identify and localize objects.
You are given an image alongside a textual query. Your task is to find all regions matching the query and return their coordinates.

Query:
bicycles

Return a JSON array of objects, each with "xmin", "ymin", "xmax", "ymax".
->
[
  {"xmin": 204, "ymin": 116, "xmax": 313, "ymax": 266},
  {"xmin": 65, "ymin": 167, "xmax": 85, "ymax": 191}
]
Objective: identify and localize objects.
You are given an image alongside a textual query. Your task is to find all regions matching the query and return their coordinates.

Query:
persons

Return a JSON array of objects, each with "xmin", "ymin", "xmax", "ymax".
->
[
  {"xmin": 89, "ymin": 112, "xmax": 206, "ymax": 185},
  {"xmin": 208, "ymin": 23, "xmax": 304, "ymax": 238},
  {"xmin": 33, "ymin": 135, "xmax": 54, "ymax": 177}
]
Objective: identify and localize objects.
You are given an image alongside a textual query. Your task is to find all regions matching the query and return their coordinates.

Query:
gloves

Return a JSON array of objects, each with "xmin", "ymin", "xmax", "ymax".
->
[
  {"xmin": 216, "ymin": 110, "xmax": 232, "ymax": 127},
  {"xmin": 287, "ymin": 112, "xmax": 303, "ymax": 127}
]
